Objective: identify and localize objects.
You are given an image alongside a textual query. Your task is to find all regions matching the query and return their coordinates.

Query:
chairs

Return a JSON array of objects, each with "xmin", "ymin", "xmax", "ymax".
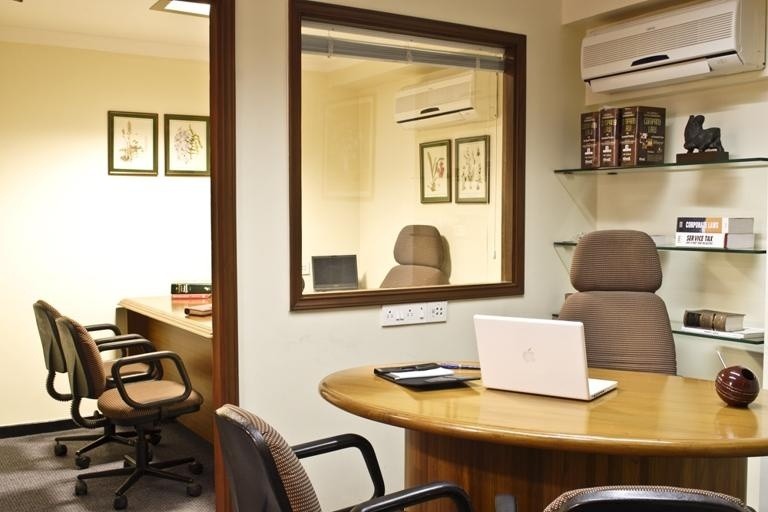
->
[
  {"xmin": 214, "ymin": 403, "xmax": 475, "ymax": 511},
  {"xmin": 33, "ymin": 301, "xmax": 164, "ymax": 468},
  {"xmin": 380, "ymin": 225, "xmax": 444, "ymax": 286},
  {"xmin": 543, "ymin": 486, "xmax": 755, "ymax": 511},
  {"xmin": 557, "ymin": 230, "xmax": 676, "ymax": 377},
  {"xmin": 56, "ymin": 316, "xmax": 203, "ymax": 509}
]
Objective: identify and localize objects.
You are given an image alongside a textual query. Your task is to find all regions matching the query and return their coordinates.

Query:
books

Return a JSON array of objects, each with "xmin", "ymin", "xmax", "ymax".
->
[
  {"xmin": 674, "ymin": 216, "xmax": 755, "ymax": 233},
  {"xmin": 680, "ymin": 309, "xmax": 767, "ymax": 340},
  {"xmin": 170, "ymin": 282, "xmax": 212, "ymax": 300},
  {"xmin": 184, "ymin": 303, "xmax": 214, "ymax": 317},
  {"xmin": 675, "ymin": 232, "xmax": 755, "ymax": 251}
]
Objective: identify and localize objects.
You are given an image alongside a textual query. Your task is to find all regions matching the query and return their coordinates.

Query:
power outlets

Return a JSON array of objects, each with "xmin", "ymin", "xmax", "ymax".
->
[{"xmin": 380, "ymin": 301, "xmax": 448, "ymax": 326}]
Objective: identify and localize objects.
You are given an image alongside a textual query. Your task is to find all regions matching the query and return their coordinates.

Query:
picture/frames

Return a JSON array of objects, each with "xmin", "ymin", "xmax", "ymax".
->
[
  {"xmin": 108, "ymin": 111, "xmax": 158, "ymax": 176},
  {"xmin": 454, "ymin": 135, "xmax": 490, "ymax": 203},
  {"xmin": 419, "ymin": 139, "xmax": 452, "ymax": 203},
  {"xmin": 164, "ymin": 114, "xmax": 210, "ymax": 177}
]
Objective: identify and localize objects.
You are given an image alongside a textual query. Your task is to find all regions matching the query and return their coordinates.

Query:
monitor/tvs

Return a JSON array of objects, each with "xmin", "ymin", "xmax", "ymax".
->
[{"xmin": 312, "ymin": 255, "xmax": 358, "ymax": 290}]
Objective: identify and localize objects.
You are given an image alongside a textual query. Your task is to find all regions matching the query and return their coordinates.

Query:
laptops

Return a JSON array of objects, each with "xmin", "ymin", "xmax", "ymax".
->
[{"xmin": 474, "ymin": 314, "xmax": 618, "ymax": 401}]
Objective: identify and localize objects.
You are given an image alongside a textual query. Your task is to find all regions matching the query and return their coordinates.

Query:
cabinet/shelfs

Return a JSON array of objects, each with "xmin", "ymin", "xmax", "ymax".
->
[{"xmin": 552, "ymin": 157, "xmax": 768, "ymax": 343}]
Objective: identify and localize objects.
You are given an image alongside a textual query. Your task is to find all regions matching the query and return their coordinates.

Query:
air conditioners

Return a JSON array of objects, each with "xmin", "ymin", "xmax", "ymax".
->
[
  {"xmin": 580, "ymin": 0, "xmax": 767, "ymax": 94},
  {"xmin": 394, "ymin": 70, "xmax": 498, "ymax": 131}
]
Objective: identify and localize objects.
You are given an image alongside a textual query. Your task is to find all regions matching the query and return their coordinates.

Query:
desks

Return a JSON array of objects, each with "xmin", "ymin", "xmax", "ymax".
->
[
  {"xmin": 116, "ymin": 296, "xmax": 213, "ymax": 448},
  {"xmin": 319, "ymin": 364, "xmax": 768, "ymax": 512}
]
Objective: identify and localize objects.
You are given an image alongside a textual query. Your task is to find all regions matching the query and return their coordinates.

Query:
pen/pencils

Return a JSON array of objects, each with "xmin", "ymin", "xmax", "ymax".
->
[{"xmin": 439, "ymin": 364, "xmax": 480, "ymax": 370}]
